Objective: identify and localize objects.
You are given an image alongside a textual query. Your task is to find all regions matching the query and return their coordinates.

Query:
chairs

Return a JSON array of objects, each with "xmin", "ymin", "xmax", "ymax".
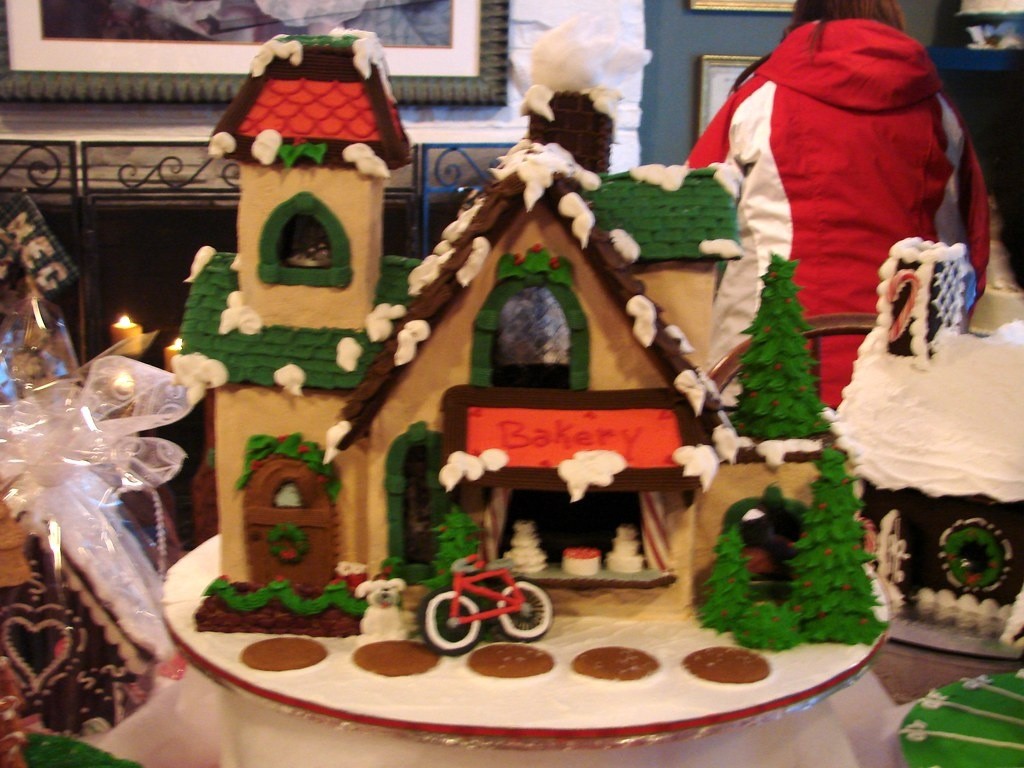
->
[{"xmin": 705, "ymin": 312, "xmax": 877, "ymax": 401}]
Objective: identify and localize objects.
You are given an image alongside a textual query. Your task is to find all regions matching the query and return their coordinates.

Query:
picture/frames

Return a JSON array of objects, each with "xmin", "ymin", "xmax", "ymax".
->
[
  {"xmin": 690, "ymin": 0, "xmax": 798, "ymax": 13},
  {"xmin": 694, "ymin": 56, "xmax": 761, "ymax": 143},
  {"xmin": 0, "ymin": 0, "xmax": 511, "ymax": 107}
]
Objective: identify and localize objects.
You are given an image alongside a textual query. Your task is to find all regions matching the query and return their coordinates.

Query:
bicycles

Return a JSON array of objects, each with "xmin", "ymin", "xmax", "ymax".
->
[{"xmin": 418, "ymin": 555, "xmax": 553, "ymax": 659}]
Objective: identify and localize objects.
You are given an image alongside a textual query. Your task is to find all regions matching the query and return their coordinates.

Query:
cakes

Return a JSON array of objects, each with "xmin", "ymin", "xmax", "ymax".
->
[{"xmin": 175, "ymin": 16, "xmax": 1024, "ymax": 662}]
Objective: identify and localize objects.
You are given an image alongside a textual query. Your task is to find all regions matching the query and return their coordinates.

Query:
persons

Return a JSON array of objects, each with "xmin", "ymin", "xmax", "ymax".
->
[{"xmin": 683, "ymin": 0, "xmax": 991, "ymax": 409}]
